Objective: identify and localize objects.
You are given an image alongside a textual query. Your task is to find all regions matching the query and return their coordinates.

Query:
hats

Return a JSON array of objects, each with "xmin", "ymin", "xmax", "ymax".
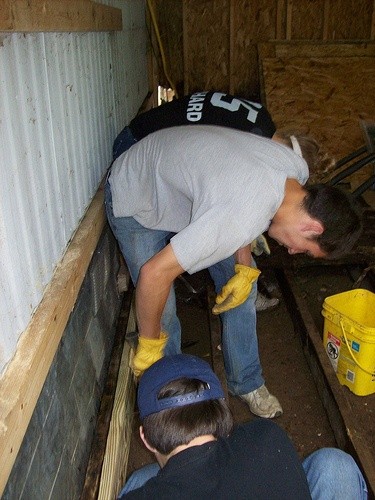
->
[{"xmin": 138, "ymin": 354, "xmax": 222, "ymax": 419}]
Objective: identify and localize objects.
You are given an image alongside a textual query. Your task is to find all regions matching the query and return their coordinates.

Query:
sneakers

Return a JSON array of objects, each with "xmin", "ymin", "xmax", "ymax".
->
[{"xmin": 238, "ymin": 385, "xmax": 284, "ymax": 418}]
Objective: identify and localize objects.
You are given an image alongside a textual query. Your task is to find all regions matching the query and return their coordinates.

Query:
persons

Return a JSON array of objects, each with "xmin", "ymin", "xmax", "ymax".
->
[
  {"xmin": 114, "ymin": 93, "xmax": 320, "ymax": 311},
  {"xmin": 121, "ymin": 352, "xmax": 367, "ymax": 500},
  {"xmin": 104, "ymin": 124, "xmax": 360, "ymax": 419}
]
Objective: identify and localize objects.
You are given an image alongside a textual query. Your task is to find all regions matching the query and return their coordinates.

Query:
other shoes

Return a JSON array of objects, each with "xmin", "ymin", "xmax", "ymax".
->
[{"xmin": 255, "ymin": 290, "xmax": 279, "ymax": 311}]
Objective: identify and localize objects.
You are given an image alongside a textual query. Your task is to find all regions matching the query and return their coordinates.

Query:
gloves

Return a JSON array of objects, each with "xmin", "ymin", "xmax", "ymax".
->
[
  {"xmin": 211, "ymin": 264, "xmax": 261, "ymax": 316},
  {"xmin": 132, "ymin": 332, "xmax": 169, "ymax": 377}
]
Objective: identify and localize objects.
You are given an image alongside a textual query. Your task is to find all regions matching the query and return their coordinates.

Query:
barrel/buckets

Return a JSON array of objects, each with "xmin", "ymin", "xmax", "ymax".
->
[{"xmin": 322, "ymin": 288, "xmax": 375, "ymax": 395}]
